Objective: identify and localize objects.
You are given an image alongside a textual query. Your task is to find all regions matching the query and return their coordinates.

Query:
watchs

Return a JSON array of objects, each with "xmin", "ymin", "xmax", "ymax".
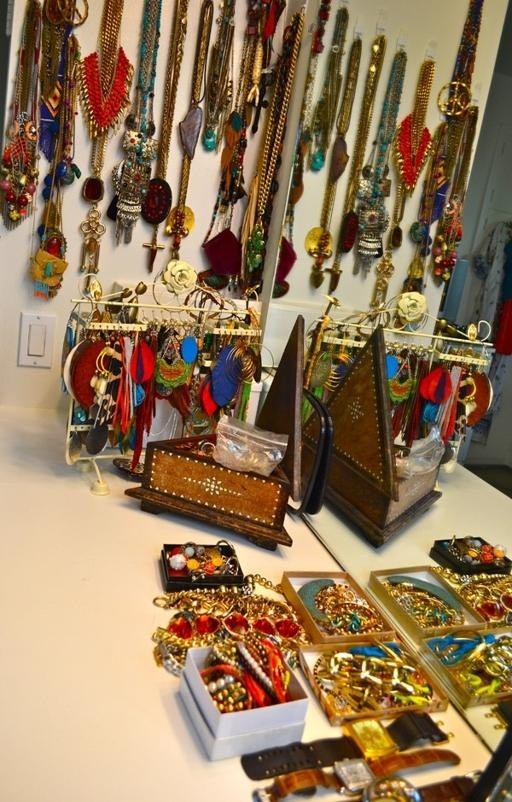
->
[{"xmin": 238, "ymin": 710, "xmax": 478, "ymax": 801}]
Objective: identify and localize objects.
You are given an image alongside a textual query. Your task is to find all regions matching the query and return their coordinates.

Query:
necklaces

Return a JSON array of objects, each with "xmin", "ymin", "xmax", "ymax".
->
[
  {"xmin": 150, "ymin": 574, "xmax": 314, "ymax": 715},
  {"xmin": 2, "ymin": 0, "xmax": 485, "ymax": 326}
]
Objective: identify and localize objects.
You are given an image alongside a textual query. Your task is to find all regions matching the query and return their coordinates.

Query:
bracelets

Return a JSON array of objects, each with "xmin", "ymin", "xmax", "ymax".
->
[{"xmin": 313, "ymin": 579, "xmax": 386, "ymax": 637}]
